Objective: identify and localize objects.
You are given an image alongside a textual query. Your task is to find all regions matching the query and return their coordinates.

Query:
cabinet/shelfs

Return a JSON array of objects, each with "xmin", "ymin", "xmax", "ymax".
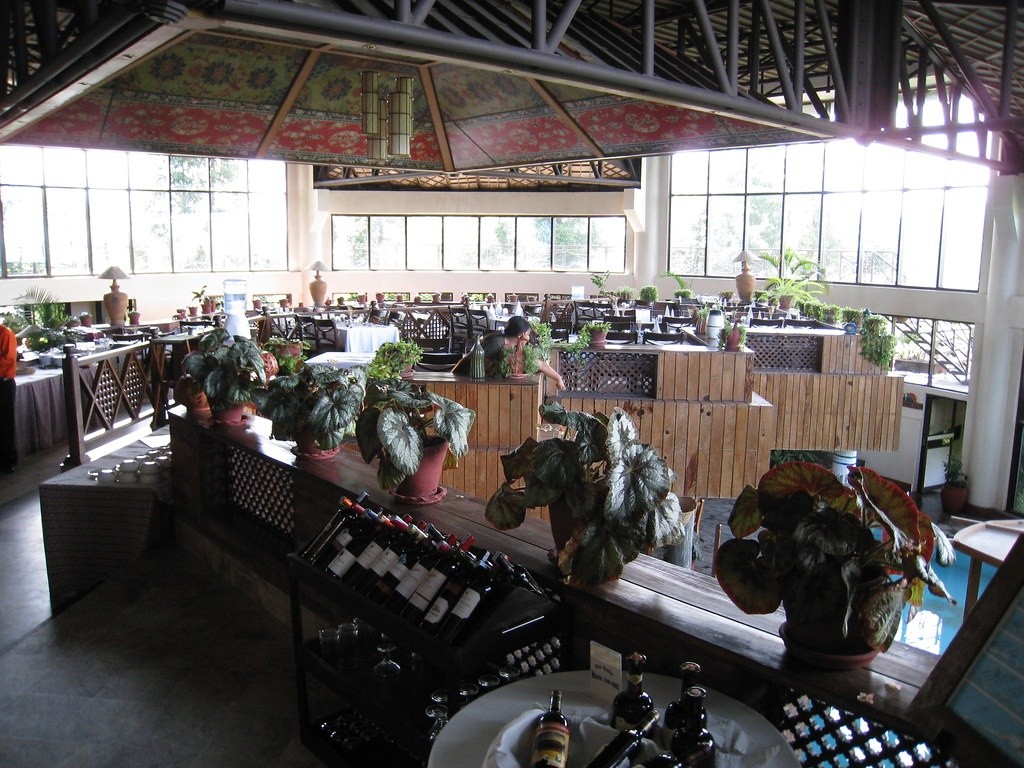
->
[{"xmin": 283, "ymin": 500, "xmax": 570, "ymax": 768}]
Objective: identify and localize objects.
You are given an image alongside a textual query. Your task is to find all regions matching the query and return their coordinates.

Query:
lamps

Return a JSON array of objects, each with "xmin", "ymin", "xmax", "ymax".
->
[
  {"xmin": 97, "ymin": 266, "xmax": 131, "ymax": 326},
  {"xmin": 302, "ymin": 260, "xmax": 333, "ymax": 305},
  {"xmin": 730, "ymin": 249, "xmax": 761, "ymax": 303}
]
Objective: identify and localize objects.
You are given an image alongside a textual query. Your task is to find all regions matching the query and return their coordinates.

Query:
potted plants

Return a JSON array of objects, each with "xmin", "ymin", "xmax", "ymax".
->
[
  {"xmin": 639, "ymin": 286, "xmax": 659, "ymax": 304},
  {"xmin": 716, "ymin": 318, "xmax": 747, "ymax": 351},
  {"xmin": 713, "ymin": 461, "xmax": 958, "ymax": 670},
  {"xmin": 614, "ymin": 285, "xmax": 639, "ymax": 302},
  {"xmin": 251, "ymin": 363, "xmax": 364, "ymax": 461},
  {"xmin": 689, "ymin": 306, "xmax": 711, "ymax": 333},
  {"xmin": 498, "ymin": 343, "xmax": 543, "ymax": 379},
  {"xmin": 486, "ymin": 293, "xmax": 494, "ymax": 303},
  {"xmin": 355, "ymin": 378, "xmax": 476, "ymax": 505},
  {"xmin": 262, "ymin": 336, "xmax": 311, "ymax": 377},
  {"xmin": 528, "ymin": 296, "xmax": 537, "ymax": 302},
  {"xmin": 65, "ymin": 285, "xmax": 470, "ymax": 329},
  {"xmin": 364, "ymin": 337, "xmax": 424, "ymax": 380},
  {"xmin": 719, "ymin": 291, "xmax": 736, "ymax": 300},
  {"xmin": 180, "ymin": 327, "xmax": 265, "ymax": 426},
  {"xmin": 557, "ymin": 320, "xmax": 611, "ymax": 363},
  {"xmin": 674, "ymin": 289, "xmax": 692, "ymax": 298},
  {"xmin": 528, "ymin": 319, "xmax": 553, "ymax": 364},
  {"xmin": 754, "ymin": 247, "xmax": 968, "ymax": 514},
  {"xmin": 483, "ymin": 401, "xmax": 684, "ymax": 586}
]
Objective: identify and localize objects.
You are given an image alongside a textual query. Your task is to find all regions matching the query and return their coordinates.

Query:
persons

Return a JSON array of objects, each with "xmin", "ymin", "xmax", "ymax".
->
[
  {"xmin": 0, "ymin": 324, "xmax": 19, "ymax": 473},
  {"xmin": 452, "ymin": 316, "xmax": 566, "ymax": 391}
]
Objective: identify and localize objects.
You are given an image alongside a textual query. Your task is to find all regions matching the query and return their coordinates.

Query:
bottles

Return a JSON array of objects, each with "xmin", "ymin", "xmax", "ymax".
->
[
  {"xmin": 367, "ymin": 529, "xmax": 439, "ymax": 606},
  {"xmin": 223, "ymin": 278, "xmax": 247, "ymax": 315},
  {"xmin": 445, "ymin": 551, "xmax": 510, "ymax": 638},
  {"xmin": 863, "ymin": 307, "xmax": 872, "ymax": 319},
  {"xmin": 496, "ymin": 554, "xmax": 544, "ymax": 596},
  {"xmin": 663, "ymin": 661, "xmax": 700, "ymax": 750},
  {"xmin": 609, "ymin": 653, "xmax": 654, "ymax": 731},
  {"xmin": 402, "ymin": 533, "xmax": 476, "ymax": 626},
  {"xmin": 632, "ymin": 743, "xmax": 711, "ymax": 768},
  {"xmin": 382, "ymin": 533, "xmax": 457, "ymax": 616},
  {"xmin": 470, "ymin": 334, "xmax": 485, "ymax": 379},
  {"xmin": 343, "ymin": 514, "xmax": 414, "ymax": 591},
  {"xmin": 350, "ymin": 520, "xmax": 428, "ymax": 597},
  {"xmin": 531, "ymin": 689, "xmax": 570, "ymax": 768},
  {"xmin": 668, "ymin": 686, "xmax": 717, "ymax": 768},
  {"xmin": 300, "ymin": 491, "xmax": 370, "ymax": 564},
  {"xmin": 418, "ymin": 548, "xmax": 490, "ymax": 638},
  {"xmin": 583, "ymin": 711, "xmax": 661, "ymax": 768},
  {"xmin": 312, "ymin": 503, "xmax": 378, "ymax": 572},
  {"xmin": 327, "ymin": 512, "xmax": 393, "ymax": 583}
]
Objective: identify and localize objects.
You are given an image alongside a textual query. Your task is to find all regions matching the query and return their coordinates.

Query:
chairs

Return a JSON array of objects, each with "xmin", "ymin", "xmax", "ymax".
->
[{"xmin": 293, "ymin": 300, "xmax": 816, "ymax": 372}]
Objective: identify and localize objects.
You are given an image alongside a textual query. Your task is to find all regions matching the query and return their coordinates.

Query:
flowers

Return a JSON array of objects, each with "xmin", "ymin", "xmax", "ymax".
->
[
  {"xmin": 397, "ymin": 292, "xmax": 403, "ymax": 296},
  {"xmin": 375, "ymin": 290, "xmax": 384, "ymax": 295},
  {"xmin": 435, "ymin": 290, "xmax": 441, "ymax": 296}
]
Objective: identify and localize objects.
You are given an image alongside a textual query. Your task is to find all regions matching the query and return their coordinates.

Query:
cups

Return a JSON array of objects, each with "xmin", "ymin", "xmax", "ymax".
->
[
  {"xmin": 98, "ymin": 441, "xmax": 171, "ymax": 484},
  {"xmin": 41, "ymin": 336, "xmax": 114, "ymax": 367},
  {"xmin": 314, "ymin": 617, "xmax": 520, "ymax": 768}
]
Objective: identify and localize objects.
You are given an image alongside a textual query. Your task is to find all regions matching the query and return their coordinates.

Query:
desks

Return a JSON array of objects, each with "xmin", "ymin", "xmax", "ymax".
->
[
  {"xmin": 305, "ymin": 352, "xmax": 377, "ymax": 371},
  {"xmin": 451, "ymin": 312, "xmax": 541, "ymax": 336},
  {"xmin": 12, "ymin": 324, "xmax": 162, "ymax": 462},
  {"xmin": 426, "ymin": 669, "xmax": 802, "ymax": 768},
  {"xmin": 567, "ymin": 335, "xmax": 693, "ymax": 394},
  {"xmin": 303, "ymin": 316, "xmax": 401, "ymax": 352},
  {"xmin": 558, "ymin": 306, "xmax": 652, "ymax": 334},
  {"xmin": 38, "ymin": 422, "xmax": 173, "ymax": 620}
]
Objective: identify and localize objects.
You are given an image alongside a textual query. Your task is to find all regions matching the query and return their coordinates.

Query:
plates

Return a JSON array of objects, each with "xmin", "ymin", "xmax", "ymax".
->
[
  {"xmin": 16, "ymin": 360, "xmax": 35, "ymax": 367},
  {"xmin": 16, "ymin": 366, "xmax": 36, "ymax": 375},
  {"xmin": 87, "ymin": 468, "xmax": 107, "ymax": 476}
]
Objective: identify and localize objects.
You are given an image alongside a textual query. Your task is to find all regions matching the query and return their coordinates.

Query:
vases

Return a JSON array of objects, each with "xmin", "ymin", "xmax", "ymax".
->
[
  {"xmin": 396, "ymin": 296, "xmax": 404, "ymax": 302},
  {"xmin": 509, "ymin": 295, "xmax": 519, "ymax": 302},
  {"xmin": 375, "ymin": 295, "xmax": 385, "ymax": 301},
  {"xmin": 431, "ymin": 295, "xmax": 439, "ymax": 302}
]
